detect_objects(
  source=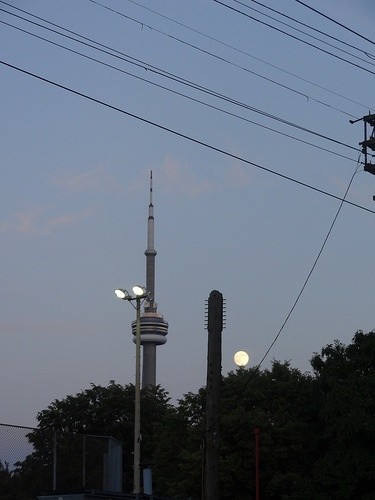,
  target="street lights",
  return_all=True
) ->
[252,424,262,499]
[113,286,152,495]
